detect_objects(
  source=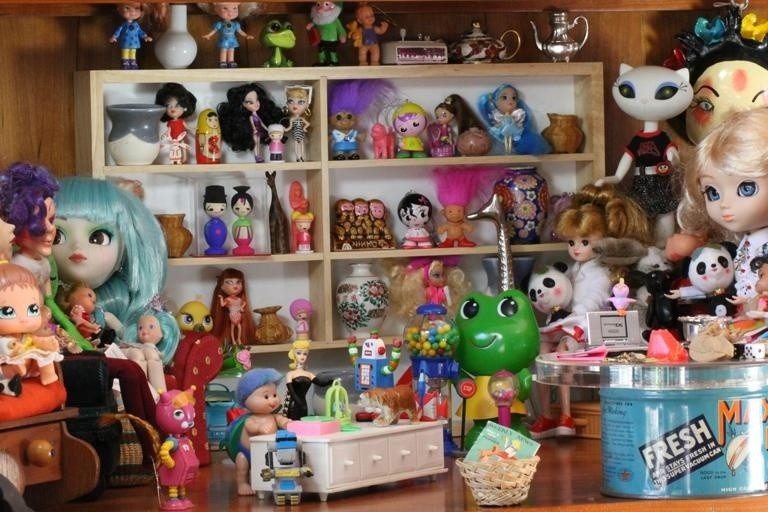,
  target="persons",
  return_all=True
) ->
[0,0,767,510]
[1,0,768,509]
